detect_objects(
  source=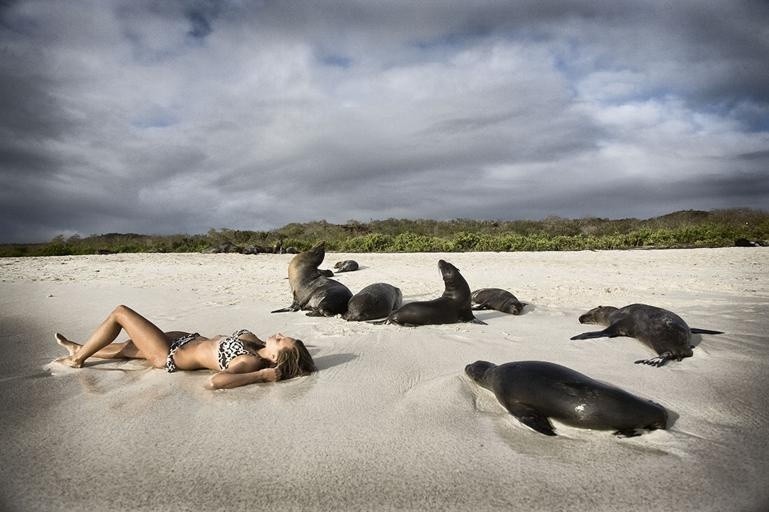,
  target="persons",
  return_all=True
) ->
[53,304,319,390]
